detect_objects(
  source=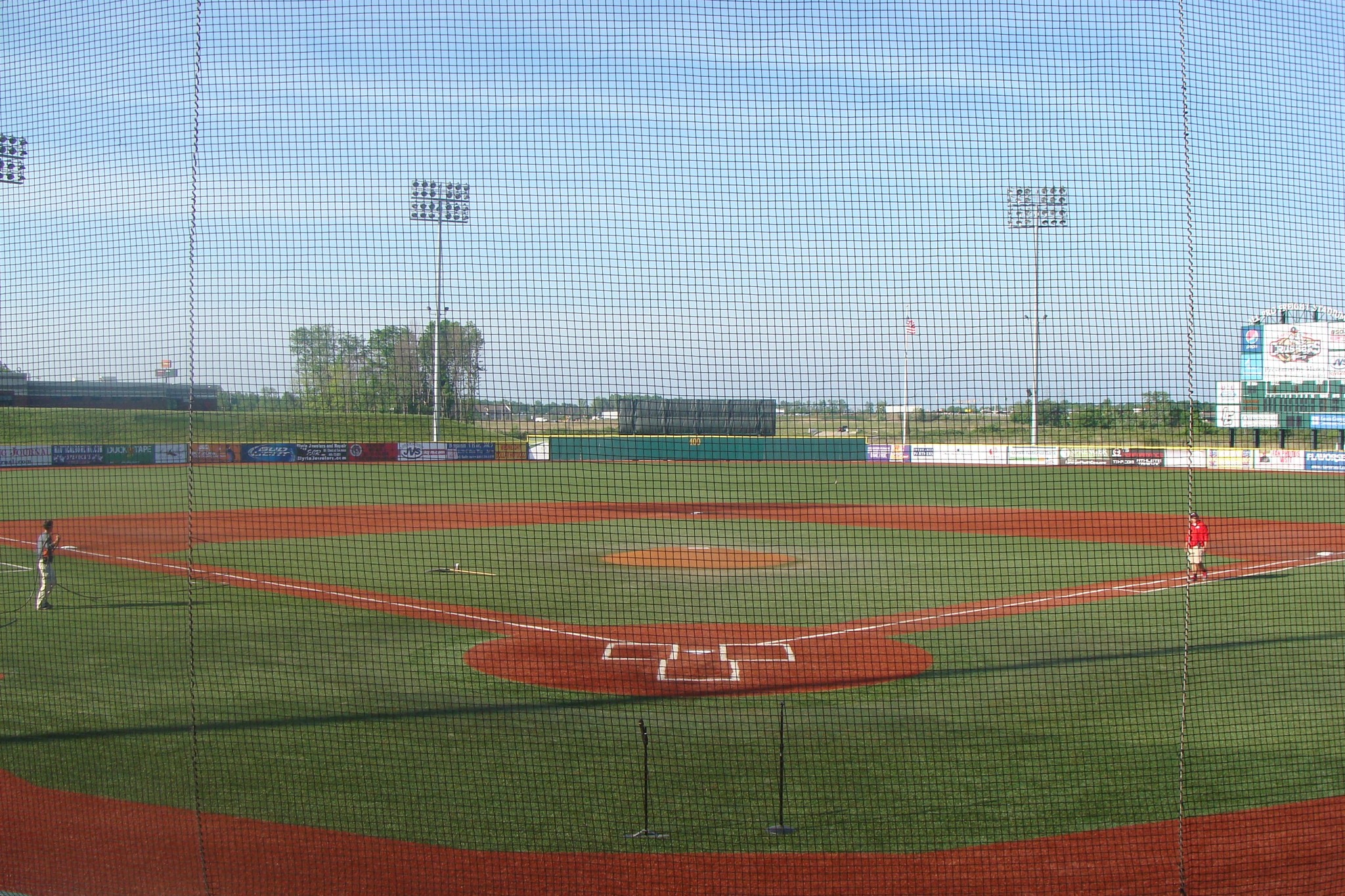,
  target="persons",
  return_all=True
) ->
[36,520,61,609]
[1184,513,1210,581]
